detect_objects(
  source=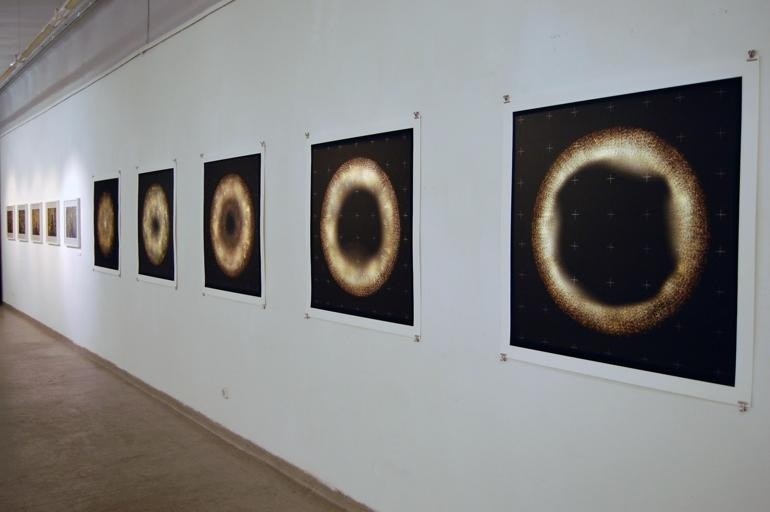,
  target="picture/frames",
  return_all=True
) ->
[6,198,82,249]
